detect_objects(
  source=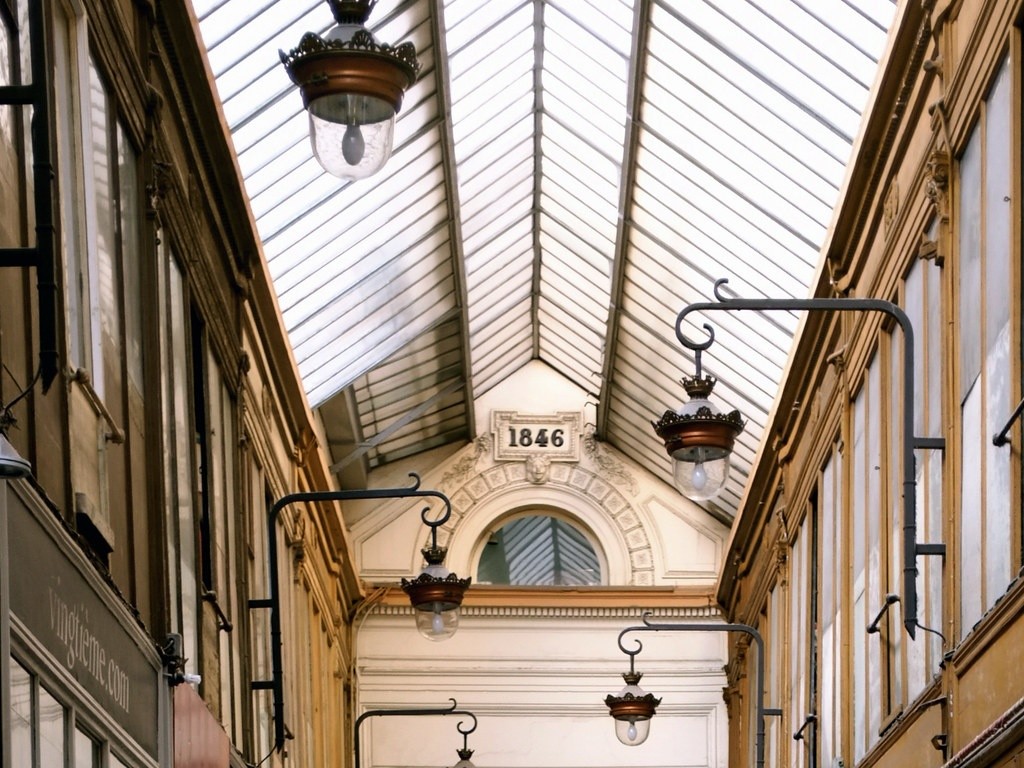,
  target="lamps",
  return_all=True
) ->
[650,286,946,642]
[604,609,785,768]
[355,698,477,768]
[243,477,474,752]
[277,3,422,181]
